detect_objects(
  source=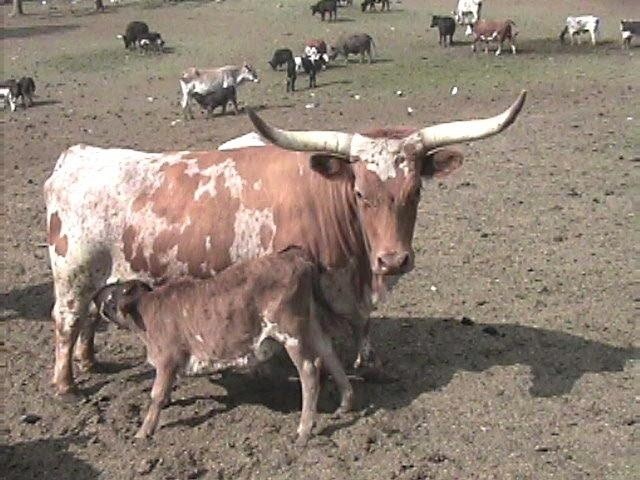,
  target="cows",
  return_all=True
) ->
[429,0,517,57]
[269,32,376,93]
[0,76,37,112]
[559,14,599,46]
[620,19,640,51]
[116,22,165,54]
[41,87,530,441]
[309,0,402,22]
[180,58,260,121]
